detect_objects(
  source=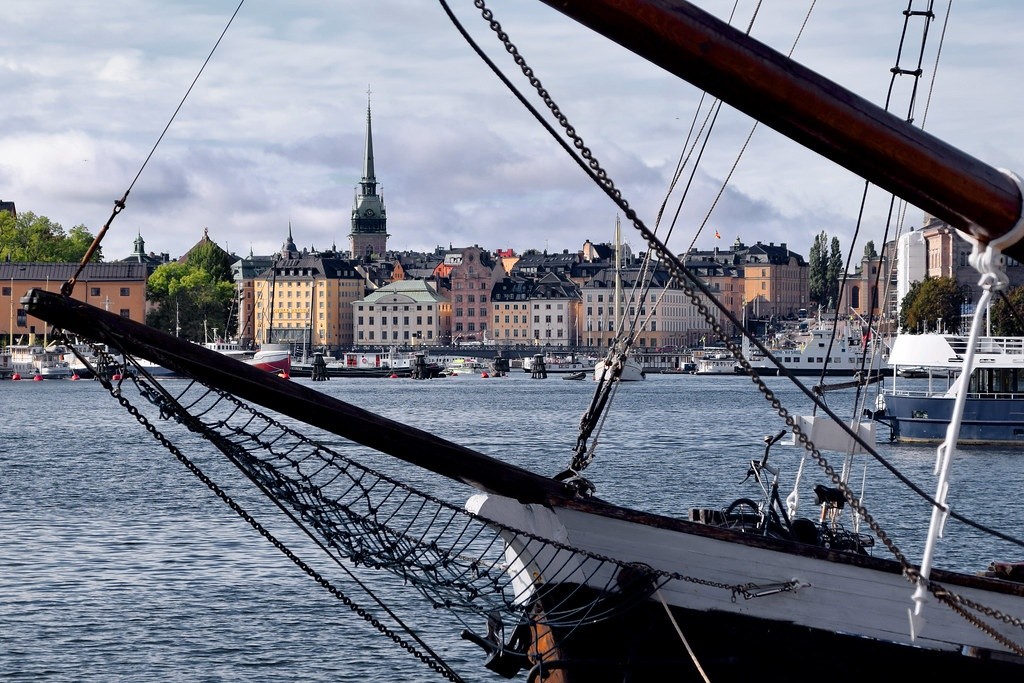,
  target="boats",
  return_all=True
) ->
[0,2,1024,683]
